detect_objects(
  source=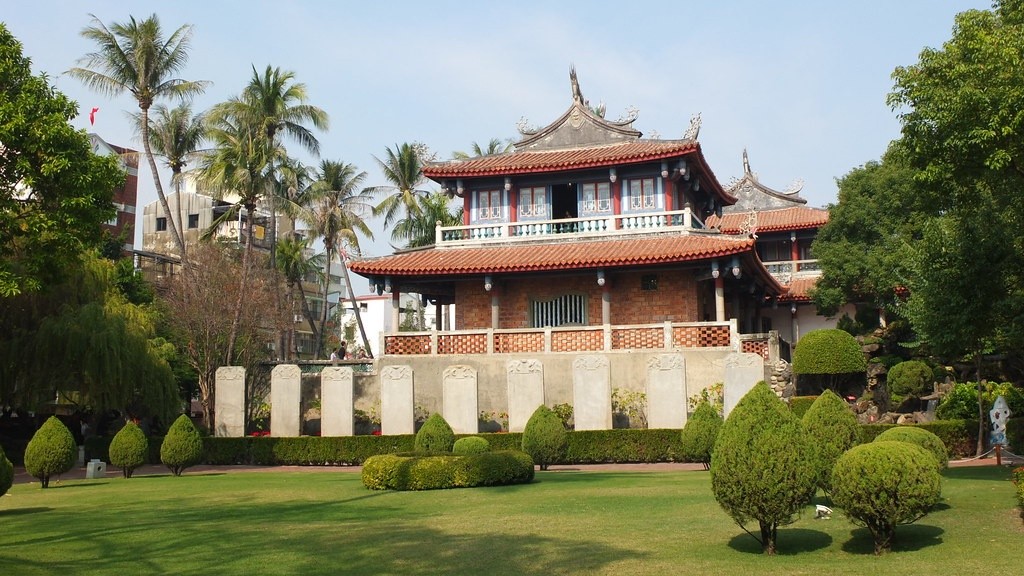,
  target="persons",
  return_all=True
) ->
[338,341,346,360]
[564,211,572,227]
[331,348,337,360]
[356,349,371,370]
